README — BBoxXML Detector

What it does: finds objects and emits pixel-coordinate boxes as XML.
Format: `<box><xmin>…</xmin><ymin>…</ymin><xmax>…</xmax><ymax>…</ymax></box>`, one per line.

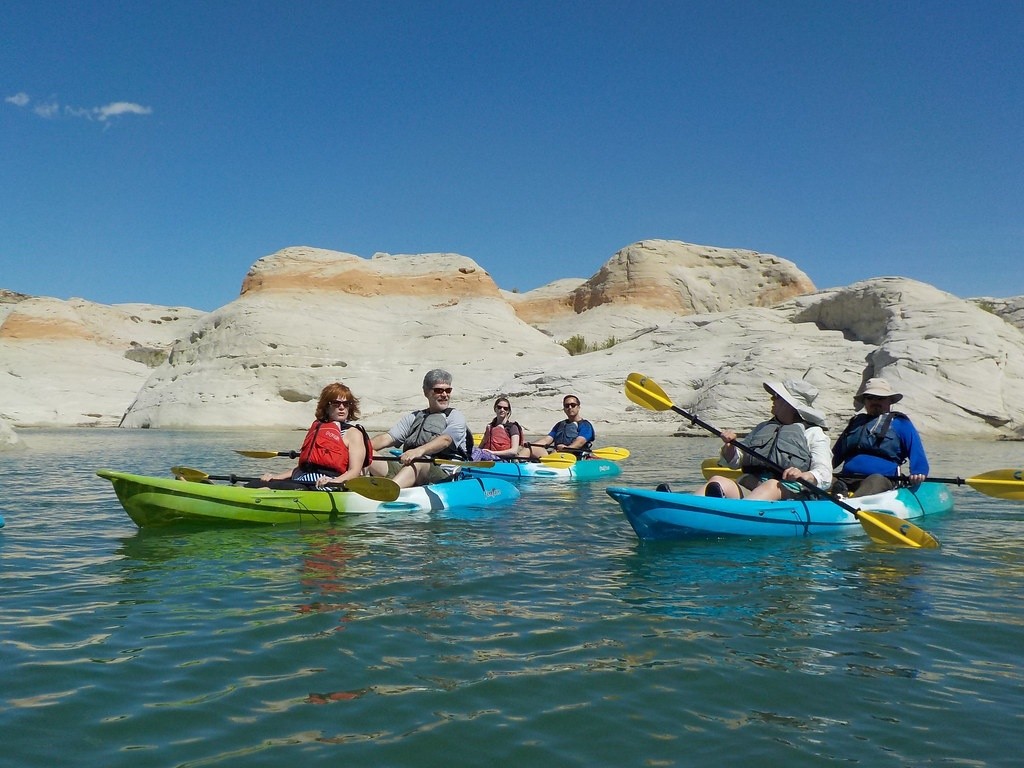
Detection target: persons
<box><xmin>656</xmin><ymin>378</ymin><xmax>833</xmax><ymax>500</ymax></box>
<box><xmin>826</xmin><ymin>379</ymin><xmax>929</xmax><ymax>499</ymax></box>
<box><xmin>174</xmin><ymin>381</ymin><xmax>374</xmax><ymax>491</ymax></box>
<box><xmin>361</xmin><ymin>367</ymin><xmax>467</xmax><ymax>489</ymax></box>
<box><xmin>512</xmin><ymin>394</ymin><xmax>595</xmax><ymax>463</ymax></box>
<box><xmin>465</xmin><ymin>398</ymin><xmax>525</xmax><ymax>462</ymax></box>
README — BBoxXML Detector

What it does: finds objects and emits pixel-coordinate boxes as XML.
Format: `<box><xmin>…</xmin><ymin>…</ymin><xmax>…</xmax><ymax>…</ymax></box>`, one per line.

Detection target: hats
<box><xmin>763</xmin><ymin>377</ymin><xmax>829</xmax><ymax>431</ymax></box>
<box><xmin>854</xmin><ymin>378</ymin><xmax>903</xmax><ymax>412</ymax></box>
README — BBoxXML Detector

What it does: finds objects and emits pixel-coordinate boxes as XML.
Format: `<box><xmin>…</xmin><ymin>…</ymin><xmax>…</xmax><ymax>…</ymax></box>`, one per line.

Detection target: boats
<box><xmin>96</xmin><ymin>463</ymin><xmax>523</xmax><ymax>532</ymax></box>
<box><xmin>604</xmin><ymin>477</ymin><xmax>955</xmax><ymax>543</ymax></box>
<box><xmin>389</xmin><ymin>439</ymin><xmax>623</xmax><ymax>483</ymax></box>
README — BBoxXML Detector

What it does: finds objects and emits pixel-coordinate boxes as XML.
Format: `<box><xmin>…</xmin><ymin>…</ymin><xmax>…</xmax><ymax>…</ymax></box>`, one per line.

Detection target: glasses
<box><xmin>497</xmin><ymin>405</ymin><xmax>508</xmax><ymax>410</ymax></box>
<box><xmin>863</xmin><ymin>394</ymin><xmax>888</xmax><ymax>400</ymax></box>
<box><xmin>429</xmin><ymin>388</ymin><xmax>452</xmax><ymax>394</ymax></box>
<box><xmin>327</xmin><ymin>400</ymin><xmax>353</xmax><ymax>407</ymax></box>
<box><xmin>563</xmin><ymin>403</ymin><xmax>578</xmax><ymax>408</ymax></box>
<box><xmin>774</xmin><ymin>393</ymin><xmax>779</xmax><ymax>399</ymax></box>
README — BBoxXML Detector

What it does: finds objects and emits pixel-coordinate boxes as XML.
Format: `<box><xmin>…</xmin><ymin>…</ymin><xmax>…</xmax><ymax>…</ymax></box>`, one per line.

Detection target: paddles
<box><xmin>624</xmin><ymin>371</ymin><xmax>938</xmax><ymax>547</ymax></box>
<box><xmin>700</xmin><ymin>459</ymin><xmax>1024</xmax><ymax>501</ymax></box>
<box><xmin>229</xmin><ymin>449</ymin><xmax>495</xmax><ymax>468</ymax></box>
<box><xmin>170</xmin><ymin>467</ymin><xmax>400</xmax><ymax>502</ymax></box>
<box><xmin>471</xmin><ymin>433</ymin><xmax>631</xmax><ymax>461</ymax></box>
<box><xmin>487</xmin><ymin>452</ymin><xmax>577</xmax><ymax>468</ymax></box>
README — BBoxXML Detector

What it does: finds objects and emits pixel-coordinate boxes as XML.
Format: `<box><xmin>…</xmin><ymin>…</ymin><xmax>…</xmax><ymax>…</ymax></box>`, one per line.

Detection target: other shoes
<box><xmin>705</xmin><ymin>482</ymin><xmax>726</xmax><ymax>498</ymax></box>
<box><xmin>656</xmin><ymin>483</ymin><xmax>671</xmax><ymax>493</ymax></box>
<box><xmin>831</xmin><ymin>480</ymin><xmax>848</xmax><ymax>500</ymax></box>
